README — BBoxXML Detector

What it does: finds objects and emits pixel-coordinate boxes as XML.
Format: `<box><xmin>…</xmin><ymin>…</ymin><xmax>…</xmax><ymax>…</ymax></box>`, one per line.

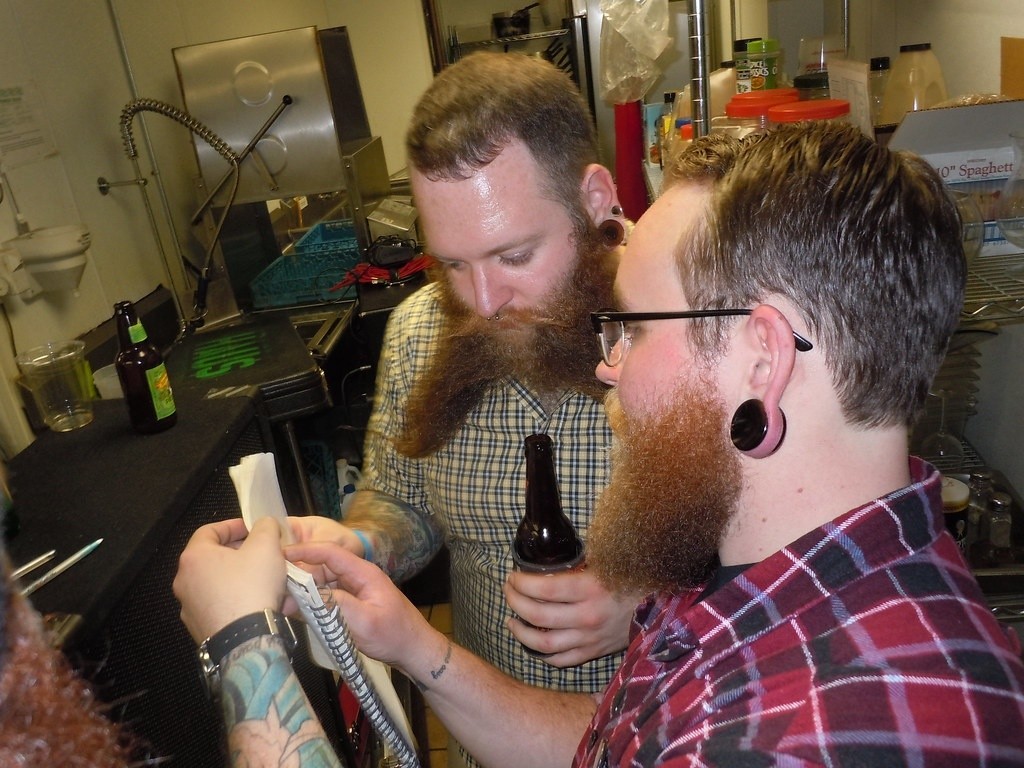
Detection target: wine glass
<box><xmin>919</xmin><ymin>383</ymin><xmax>967</xmax><ymax>472</ymax></box>
<box><xmin>994</xmin><ymin>131</ymin><xmax>1024</xmax><ymax>282</ymax></box>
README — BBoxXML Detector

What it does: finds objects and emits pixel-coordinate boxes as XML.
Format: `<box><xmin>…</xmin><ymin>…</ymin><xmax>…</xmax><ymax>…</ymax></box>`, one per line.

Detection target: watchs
<box><xmin>194</xmin><ymin>607</ymin><xmax>297</xmax><ymax>704</ymax></box>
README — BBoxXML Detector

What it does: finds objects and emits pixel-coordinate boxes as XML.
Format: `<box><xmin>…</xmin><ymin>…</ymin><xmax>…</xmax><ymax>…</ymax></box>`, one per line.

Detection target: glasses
<box><xmin>590</xmin><ymin>307</ymin><xmax>814</xmax><ymax>367</ymax></box>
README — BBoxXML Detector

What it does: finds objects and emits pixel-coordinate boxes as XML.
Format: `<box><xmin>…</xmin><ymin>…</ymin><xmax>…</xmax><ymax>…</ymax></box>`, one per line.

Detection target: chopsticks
<box><xmin>9</xmin><ymin>538</ymin><xmax>103</xmax><ymax>597</ymax></box>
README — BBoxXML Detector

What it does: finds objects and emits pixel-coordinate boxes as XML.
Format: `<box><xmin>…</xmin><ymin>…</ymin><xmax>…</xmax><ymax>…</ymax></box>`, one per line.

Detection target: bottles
<box><xmin>867</xmin><ymin>57</ymin><xmax>893</xmax><ymax>126</ymax></box>
<box><xmin>677</xmin><ymin>124</ymin><xmax>693</xmax><ymax>160</ymax></box>
<box><xmin>672</xmin><ymin>119</ymin><xmax>696</xmax><ymax>166</ymax></box>
<box><xmin>113</xmin><ymin>300</ymin><xmax>177</xmax><ymax>435</ymax></box>
<box><xmin>881</xmin><ymin>42</ymin><xmax>949</xmax><ymax>125</ymax></box>
<box><xmin>966</xmin><ymin>469</ymin><xmax>995</xmax><ymax>524</ymax></box>
<box><xmin>511</xmin><ymin>434</ymin><xmax>586</xmax><ymax>660</ymax></box>
<box><xmin>656</xmin><ymin>90</ymin><xmax>677</xmax><ymax>171</ymax></box>
<box><xmin>983</xmin><ymin>492</ymin><xmax>1012</xmax><ymax>548</ymax></box>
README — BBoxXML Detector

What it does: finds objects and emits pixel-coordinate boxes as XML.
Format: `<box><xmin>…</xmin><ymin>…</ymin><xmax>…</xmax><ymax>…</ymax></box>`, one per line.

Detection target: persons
<box><xmin>256</xmin><ymin>49</ymin><xmax>653</xmax><ymax>768</ymax></box>
<box><xmin>171</xmin><ymin>117</ymin><xmax>1024</xmax><ymax>768</ymax></box>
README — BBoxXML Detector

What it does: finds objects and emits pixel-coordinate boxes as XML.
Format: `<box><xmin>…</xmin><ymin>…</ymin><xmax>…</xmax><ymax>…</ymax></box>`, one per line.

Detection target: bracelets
<box><xmin>352</xmin><ymin>530</ymin><xmax>374</xmax><ymax>561</ymax></box>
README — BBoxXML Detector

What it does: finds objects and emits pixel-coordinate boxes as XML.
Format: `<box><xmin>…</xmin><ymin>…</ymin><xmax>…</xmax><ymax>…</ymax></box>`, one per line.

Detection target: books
<box><xmin>226</xmin><ymin>453</ymin><xmax>421</xmax><ymax>767</ymax></box>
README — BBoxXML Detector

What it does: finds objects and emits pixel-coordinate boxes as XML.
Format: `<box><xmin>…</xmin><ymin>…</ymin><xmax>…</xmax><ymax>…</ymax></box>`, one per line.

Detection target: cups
<box><xmin>14</xmin><ymin>340</ymin><xmax>94</xmax><ymax>432</ymax></box>
<box><xmin>946</xmin><ymin>186</ymin><xmax>986</xmax><ymax>263</ymax></box>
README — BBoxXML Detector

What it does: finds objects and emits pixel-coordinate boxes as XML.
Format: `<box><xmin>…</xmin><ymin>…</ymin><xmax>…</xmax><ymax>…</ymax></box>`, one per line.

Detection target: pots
<box><xmin>491</xmin><ymin>2</ymin><xmax>540</xmax><ymax>37</ymax></box>
<box><xmin>527</xmin><ymin>37</ymin><xmax>577</xmax><ymax>80</ymax></box>
